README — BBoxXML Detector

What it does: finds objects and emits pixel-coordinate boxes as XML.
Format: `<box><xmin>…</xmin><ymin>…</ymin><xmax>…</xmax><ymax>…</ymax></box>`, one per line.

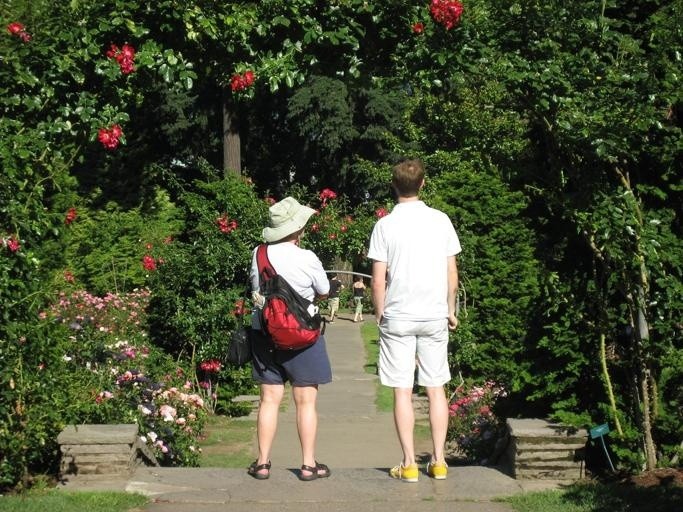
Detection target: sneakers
<box><xmin>389</xmin><ymin>463</ymin><xmax>418</xmax><ymax>483</ymax></box>
<box><xmin>425</xmin><ymin>462</ymin><xmax>447</xmax><ymax>481</ymax></box>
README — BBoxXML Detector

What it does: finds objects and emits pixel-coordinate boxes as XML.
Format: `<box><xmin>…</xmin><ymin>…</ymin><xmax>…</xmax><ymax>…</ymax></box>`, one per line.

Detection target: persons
<box><xmin>350</xmin><ymin>276</ymin><xmax>366</xmax><ymax>323</ymax></box>
<box><xmin>327</xmin><ymin>273</ymin><xmax>345</xmax><ymax>324</ymax></box>
<box><xmin>367</xmin><ymin>159</ymin><xmax>463</xmax><ymax>482</ymax></box>
<box><xmin>247</xmin><ymin>196</ymin><xmax>332</xmax><ymax>481</ymax></box>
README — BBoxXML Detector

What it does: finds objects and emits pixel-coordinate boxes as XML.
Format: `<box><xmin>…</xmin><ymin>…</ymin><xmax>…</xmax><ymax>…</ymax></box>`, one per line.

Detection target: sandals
<box><xmin>301</xmin><ymin>461</ymin><xmax>331</xmax><ymax>481</ymax></box>
<box><xmin>248</xmin><ymin>459</ymin><xmax>271</xmax><ymax>480</ymax></box>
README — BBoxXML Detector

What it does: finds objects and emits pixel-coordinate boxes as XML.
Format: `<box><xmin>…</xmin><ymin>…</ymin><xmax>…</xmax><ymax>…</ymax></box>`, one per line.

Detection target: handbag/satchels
<box><xmin>258</xmin><ymin>275</ymin><xmax>320</xmax><ymax>351</ymax></box>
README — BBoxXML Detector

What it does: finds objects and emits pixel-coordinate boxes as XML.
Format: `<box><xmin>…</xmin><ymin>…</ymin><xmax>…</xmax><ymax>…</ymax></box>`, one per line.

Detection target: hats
<box><xmin>261</xmin><ymin>196</ymin><xmax>317</xmax><ymax>242</ymax></box>
